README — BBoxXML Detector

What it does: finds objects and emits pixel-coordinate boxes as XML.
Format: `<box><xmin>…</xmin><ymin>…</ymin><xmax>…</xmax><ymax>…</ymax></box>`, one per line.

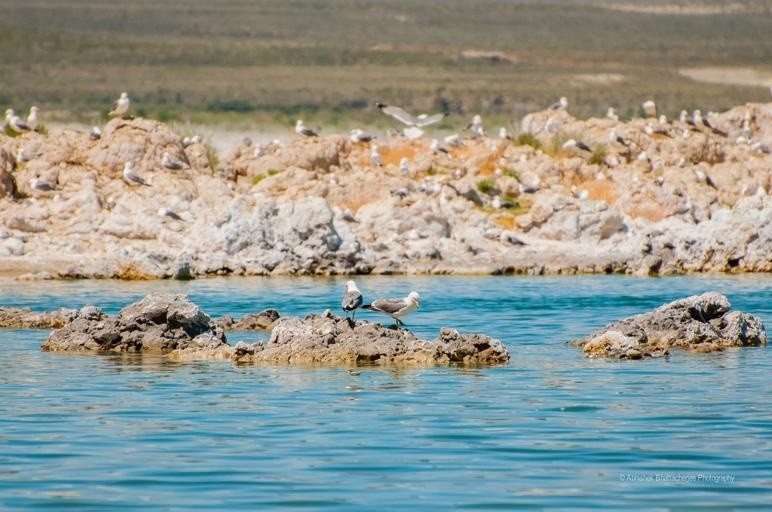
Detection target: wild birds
<box><xmin>341</xmin><ymin>279</ymin><xmax>362</xmax><ymax>324</ymax></box>
<box><xmin>5</xmin><ymin>91</ymin><xmax>770</xmax><ymax>223</ymax></box>
<box><xmin>358</xmin><ymin>290</ymin><xmax>422</xmax><ymax>326</ymax></box>
